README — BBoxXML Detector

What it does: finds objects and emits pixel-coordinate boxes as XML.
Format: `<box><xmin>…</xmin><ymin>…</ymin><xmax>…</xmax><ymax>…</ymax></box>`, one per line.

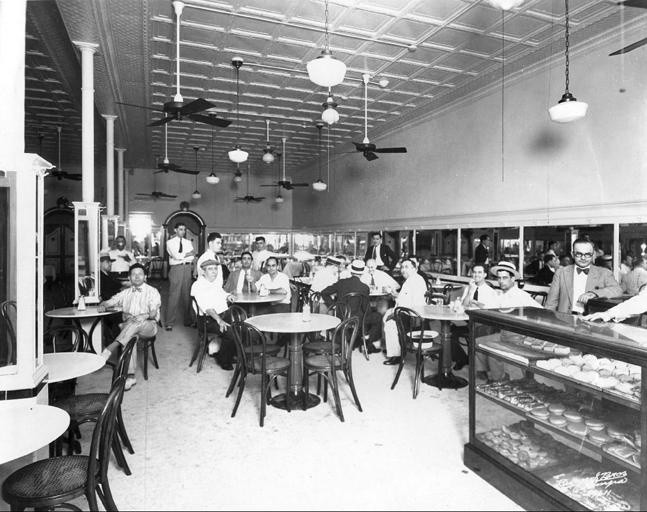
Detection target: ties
<box><xmin>242</xmin><ymin>274</ymin><xmax>248</xmax><ymax>295</ymax></box>
<box><xmin>474</xmin><ymin>288</ymin><xmax>478</xmax><ymax>300</ymax></box>
<box><xmin>373</xmin><ymin>247</ymin><xmax>376</xmax><ymax>258</ymax></box>
<box><xmin>179</xmin><ymin>240</ymin><xmax>182</xmax><ymax>252</ymax></box>
<box><xmin>371</xmin><ymin>274</ymin><xmax>374</xmax><ymax>285</ymax></box>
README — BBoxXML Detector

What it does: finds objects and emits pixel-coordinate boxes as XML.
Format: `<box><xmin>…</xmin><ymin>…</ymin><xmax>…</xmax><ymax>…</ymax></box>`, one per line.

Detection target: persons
<box><xmin>96</xmin><ymin>262</ymin><xmax>162</xmax><ymax>392</ymax></box>
<box><xmin>190</xmin><ymin>232</ymin><xmax>647</xmax><ymax>403</ymax></box>
<box><xmin>109</xmin><ymin>235</ymin><xmax>137</xmax><ymax>275</ymax></box>
<box><xmin>164</xmin><ymin>221</ymin><xmax>195</xmax><ymax>331</ymax></box>
<box><xmin>472</xmin><ymin>234</ymin><xmax>647</xmax><ymax>294</ymax></box>
<box><xmin>99</xmin><ymin>251</ymin><xmax>123</xmax><ymax>301</ymax></box>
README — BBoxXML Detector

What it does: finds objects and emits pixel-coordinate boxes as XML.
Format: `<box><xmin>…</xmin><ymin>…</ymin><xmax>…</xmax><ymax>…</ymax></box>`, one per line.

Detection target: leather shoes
<box><xmin>125</xmin><ymin>378</ymin><xmax>137</xmax><ymax>391</ymax></box>
<box><xmin>220</xmin><ymin>363</ymin><xmax>233</xmax><ymax>370</ymax></box>
<box><xmin>368</xmin><ymin>348</ymin><xmax>510</xmax><ymax>383</ymax></box>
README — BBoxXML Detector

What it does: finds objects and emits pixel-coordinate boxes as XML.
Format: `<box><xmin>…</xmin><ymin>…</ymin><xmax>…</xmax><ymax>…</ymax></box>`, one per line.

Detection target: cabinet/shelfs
<box><xmin>462</xmin><ymin>323</ymin><xmax>647</xmax><ymax>512</ymax></box>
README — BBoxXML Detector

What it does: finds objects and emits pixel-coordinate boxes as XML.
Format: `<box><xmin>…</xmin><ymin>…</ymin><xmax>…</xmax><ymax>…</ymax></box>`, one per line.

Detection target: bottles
<box><xmin>303</xmin><ymin>303</ymin><xmax>312</xmax><ymax>322</ymax></box>
<box><xmin>77</xmin><ymin>295</ymin><xmax>86</xmax><ymax>311</ymax></box>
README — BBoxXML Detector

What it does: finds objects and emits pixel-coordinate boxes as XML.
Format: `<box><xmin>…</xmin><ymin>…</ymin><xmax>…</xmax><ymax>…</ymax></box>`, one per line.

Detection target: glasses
<box><xmin>573</xmin><ymin>252</ymin><xmax>594</xmax><ymax>258</ymax></box>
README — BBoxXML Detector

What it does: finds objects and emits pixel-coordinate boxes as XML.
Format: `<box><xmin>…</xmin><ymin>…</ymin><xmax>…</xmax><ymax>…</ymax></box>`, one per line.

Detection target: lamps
<box><xmin>485</xmin><ymin>1</ymin><xmax>588</xmax><ymax>123</ymax></box>
<box><xmin>306</xmin><ymin>0</ymin><xmax>346</xmax><ymax>191</ymax></box>
<box><xmin>191</xmin><ymin>128</ymin><xmax>220</xmax><ymax>199</ymax></box>
<box><xmin>227</xmin><ymin>56</ymin><xmax>276</xmax><ymax>164</ymax></box>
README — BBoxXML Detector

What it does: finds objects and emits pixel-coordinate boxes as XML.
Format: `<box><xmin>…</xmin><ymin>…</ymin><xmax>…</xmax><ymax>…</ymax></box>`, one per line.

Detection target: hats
<box><xmin>100</xmin><ymin>252</ymin><xmax>116</xmax><ymax>262</ymax></box>
<box><xmin>491</xmin><ymin>261</ymin><xmax>521</xmax><ymax>281</ymax></box>
<box><xmin>200</xmin><ymin>260</ymin><xmax>221</xmax><ymax>266</ymax></box>
<box><xmin>326</xmin><ymin>256</ymin><xmax>342</xmax><ymax>266</ymax></box>
<box><xmin>347</xmin><ymin>259</ymin><xmax>369</xmax><ymax>274</ymax></box>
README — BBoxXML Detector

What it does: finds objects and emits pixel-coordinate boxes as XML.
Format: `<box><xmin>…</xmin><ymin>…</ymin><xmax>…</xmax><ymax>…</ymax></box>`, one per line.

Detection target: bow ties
<box><xmin>132</xmin><ymin>287</ymin><xmax>142</xmax><ymax>292</ymax></box>
<box><xmin>577</xmin><ymin>267</ymin><xmax>590</xmax><ymax>274</ymax></box>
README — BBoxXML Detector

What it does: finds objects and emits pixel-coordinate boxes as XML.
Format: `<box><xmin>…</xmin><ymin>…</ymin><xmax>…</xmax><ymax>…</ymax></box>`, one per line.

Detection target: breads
<box><xmin>530</xmin><ymin>399</ymin><xmax>642</xmax><ymax>469</ymax></box>
<box><xmin>544</xmin><ymin>466</ymin><xmax>644</xmax><ymax>512</ymax></box>
<box><xmin>473</xmin><ymin>422</ymin><xmax>581</xmax><ymax>471</ymax></box>
<box><xmin>475</xmin><ymin>376</ymin><xmax>567</xmax><ymax>411</ymax></box>
<box><xmin>500</xmin><ymin>329</ymin><xmax>571</xmax><ymax>356</ymax></box>
<box><xmin>535</xmin><ymin>354</ymin><xmax>642</xmax><ymax>399</ymax></box>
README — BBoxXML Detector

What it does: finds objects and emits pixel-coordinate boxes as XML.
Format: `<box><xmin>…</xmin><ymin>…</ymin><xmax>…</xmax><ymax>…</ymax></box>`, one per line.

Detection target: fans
<box><xmin>610</xmin><ymin>1</ymin><xmax>647</xmax><ymax>55</ymax></box>
<box><xmin>112</xmin><ymin>1</ymin><xmax>233</xmax><ymax>128</ymax></box>
<box><xmin>260</xmin><ymin>136</ymin><xmax>309</xmax><ymax>191</ymax></box>
<box><xmin>135</xmin><ymin>191</ymin><xmax>177</xmax><ymax>199</ymax></box>
<box><xmin>234</xmin><ymin>164</ymin><xmax>266</xmax><ymax>202</ymax></box>
<box><xmin>333</xmin><ymin>73</ymin><xmax>408</xmax><ymax>160</ymax></box>
<box><xmin>151</xmin><ymin>112</ymin><xmax>200</xmax><ymax>175</ymax></box>
<box><xmin>50</xmin><ymin>124</ymin><xmax>82</xmax><ymax>180</ymax></box>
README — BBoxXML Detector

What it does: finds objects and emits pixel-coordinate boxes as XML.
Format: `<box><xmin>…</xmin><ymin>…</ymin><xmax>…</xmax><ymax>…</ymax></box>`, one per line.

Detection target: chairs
<box><xmin>1</xmin><ymin>299</ymin><xmax>159</xmax><ymax>511</ymax></box>
